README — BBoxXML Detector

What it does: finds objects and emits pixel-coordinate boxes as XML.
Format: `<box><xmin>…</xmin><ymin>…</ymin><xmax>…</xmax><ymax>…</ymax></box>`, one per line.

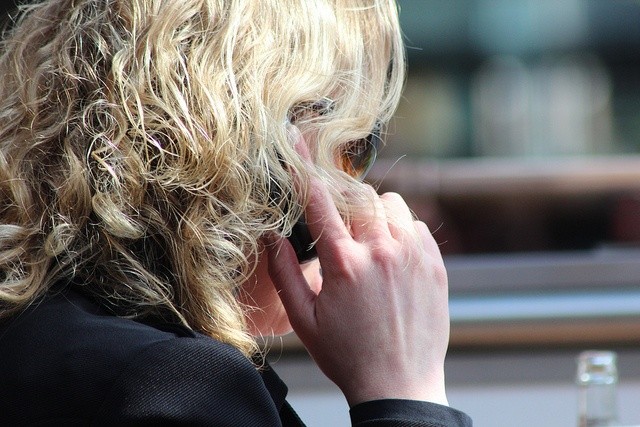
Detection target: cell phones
<box><xmin>242</xmin><ymin>144</ymin><xmax>318</xmax><ymax>271</ymax></box>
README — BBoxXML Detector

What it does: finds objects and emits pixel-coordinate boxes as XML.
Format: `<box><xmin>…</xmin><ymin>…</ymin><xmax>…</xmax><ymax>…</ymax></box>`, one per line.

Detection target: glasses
<box><xmin>339</xmin><ymin>132</ymin><xmax>378</xmax><ymax>180</ymax></box>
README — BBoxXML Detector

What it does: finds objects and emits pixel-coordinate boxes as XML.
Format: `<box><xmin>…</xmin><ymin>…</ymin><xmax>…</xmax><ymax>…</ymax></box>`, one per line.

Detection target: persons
<box><xmin>0</xmin><ymin>0</ymin><xmax>472</xmax><ymax>427</ymax></box>
